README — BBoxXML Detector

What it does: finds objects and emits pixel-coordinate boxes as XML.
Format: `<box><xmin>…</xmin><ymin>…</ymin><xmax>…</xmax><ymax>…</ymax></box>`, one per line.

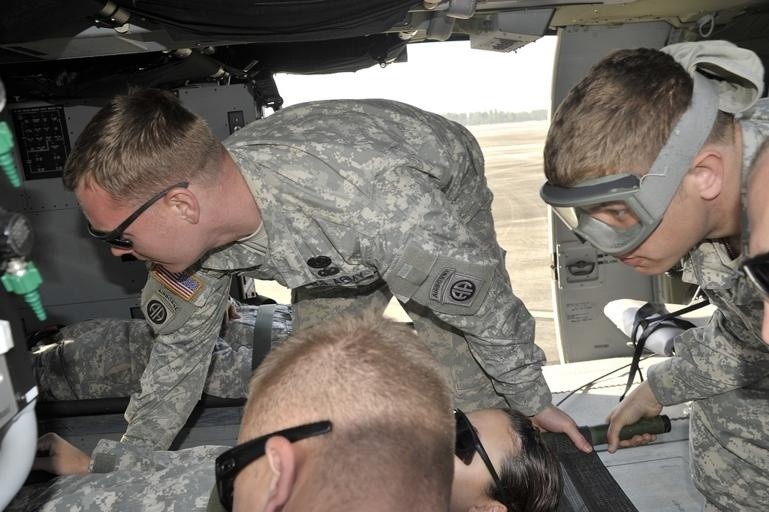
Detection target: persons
<box><xmin>746</xmin><ymin>136</ymin><xmax>769</xmax><ymax>346</ymax></box>
<box><xmin>230</xmin><ymin>312</ymin><xmax>455</xmax><ymax>512</ymax></box>
<box><xmin>543</xmin><ymin>48</ymin><xmax>769</xmax><ymax>512</ymax></box>
<box><xmin>28</xmin><ymin>294</ymin><xmax>297</xmax><ymax>402</ymax></box>
<box><xmin>60</xmin><ymin>86</ymin><xmax>592</xmax><ymax>454</ymax></box>
<box><xmin>2</xmin><ymin>409</ymin><xmax>563</xmax><ymax>512</ymax></box>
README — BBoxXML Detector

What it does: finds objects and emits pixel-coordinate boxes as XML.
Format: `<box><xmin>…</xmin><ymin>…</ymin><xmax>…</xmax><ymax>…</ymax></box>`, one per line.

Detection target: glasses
<box><xmin>215</xmin><ymin>421</ymin><xmax>332</xmax><ymax>512</ymax></box>
<box><xmin>455</xmin><ymin>408</ymin><xmax>508</xmax><ymax>502</ymax></box>
<box><xmin>85</xmin><ymin>182</ymin><xmax>190</xmax><ymax>252</ymax></box>
<box><xmin>540</xmin><ymin>129</ymin><xmax>710</xmax><ymax>260</ymax></box>
